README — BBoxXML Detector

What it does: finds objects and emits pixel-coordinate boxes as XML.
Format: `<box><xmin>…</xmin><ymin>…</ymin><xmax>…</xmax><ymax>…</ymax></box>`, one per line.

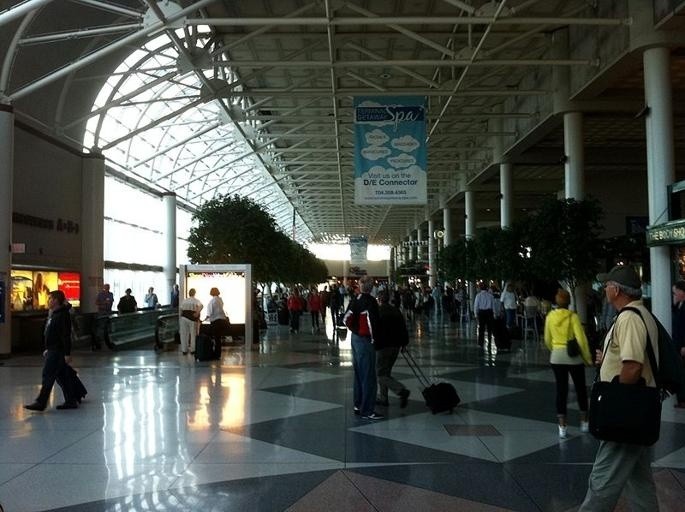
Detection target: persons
<box><xmin>171</xmin><ymin>284</ymin><xmax>179</xmax><ymax>309</ymax></box>
<box><xmin>672</xmin><ymin>283</ymin><xmax>685</xmax><ymax>408</ymax></box>
<box><xmin>145</xmin><ymin>287</ymin><xmax>158</xmax><ymax>307</ymax></box>
<box><xmin>95</xmin><ymin>284</ymin><xmax>114</xmax><ymax>313</ymax></box>
<box><xmin>577</xmin><ymin>263</ymin><xmax>660</xmax><ymax>511</ymax></box>
<box><xmin>178</xmin><ymin>289</ymin><xmax>203</xmax><ymax>355</ymax></box>
<box><xmin>252</xmin><ymin>264</ymin><xmax>618</xmax><ymax>439</ymax></box>
<box><xmin>23</xmin><ymin>290</ymin><xmax>87</xmax><ymax>411</ymax></box>
<box><xmin>117</xmin><ymin>289</ymin><xmax>137</xmax><ymax>314</ymax></box>
<box><xmin>200</xmin><ymin>288</ymin><xmax>227</xmax><ymax>360</ymax></box>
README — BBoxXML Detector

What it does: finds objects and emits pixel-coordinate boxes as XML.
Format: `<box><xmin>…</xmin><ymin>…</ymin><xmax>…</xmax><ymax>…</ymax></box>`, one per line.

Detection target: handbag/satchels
<box><xmin>589</xmin><ymin>375</ymin><xmax>661</xmax><ymax>446</ymax></box>
<box><xmin>567</xmin><ymin>339</ymin><xmax>580</xmax><ymax>358</ymax></box>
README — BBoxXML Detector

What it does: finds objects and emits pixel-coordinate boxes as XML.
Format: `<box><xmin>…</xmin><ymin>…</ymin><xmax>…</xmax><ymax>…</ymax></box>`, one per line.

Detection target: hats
<box><xmin>596</xmin><ymin>266</ymin><xmax>640</xmax><ymax>288</ymax></box>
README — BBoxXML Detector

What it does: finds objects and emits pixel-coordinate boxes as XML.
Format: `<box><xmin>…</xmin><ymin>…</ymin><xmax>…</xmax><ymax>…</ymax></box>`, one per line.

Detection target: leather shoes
<box><xmin>24</xmin><ymin>403</ymin><xmax>45</xmax><ymax>411</ymax></box>
<box><xmin>56</xmin><ymin>402</ymin><xmax>77</xmax><ymax>409</ymax></box>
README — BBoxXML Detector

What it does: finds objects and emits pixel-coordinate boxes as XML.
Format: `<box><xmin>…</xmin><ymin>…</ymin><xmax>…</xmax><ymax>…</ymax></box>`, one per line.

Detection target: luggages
<box><xmin>195</xmin><ymin>334</ymin><xmax>212</xmax><ymax>361</ymax></box>
<box><xmin>57</xmin><ymin>365</ymin><xmax>87</xmax><ymax>404</ymax></box>
<box><xmin>401</xmin><ymin>349</ymin><xmax>460</xmax><ymax>413</ymax></box>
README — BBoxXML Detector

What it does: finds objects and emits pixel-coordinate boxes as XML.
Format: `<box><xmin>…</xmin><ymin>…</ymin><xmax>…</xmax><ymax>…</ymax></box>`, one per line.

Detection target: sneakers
<box><xmin>559</xmin><ymin>421</ymin><xmax>588</xmax><ymax>438</ymax></box>
<box><xmin>353</xmin><ymin>390</ymin><xmax>410</xmax><ymax>420</ymax></box>
